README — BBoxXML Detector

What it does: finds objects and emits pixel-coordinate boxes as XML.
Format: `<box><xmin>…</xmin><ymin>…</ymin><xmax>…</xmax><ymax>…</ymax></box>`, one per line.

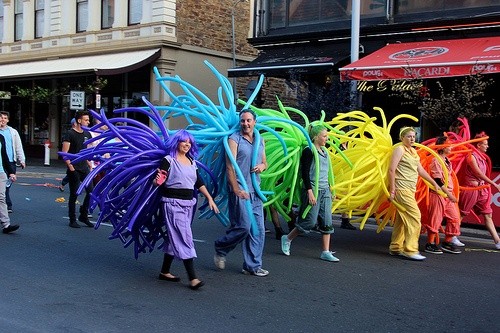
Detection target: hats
<box><xmin>71</xmin><ymin>118</ymin><xmax>76</xmax><ymax>124</ymax></box>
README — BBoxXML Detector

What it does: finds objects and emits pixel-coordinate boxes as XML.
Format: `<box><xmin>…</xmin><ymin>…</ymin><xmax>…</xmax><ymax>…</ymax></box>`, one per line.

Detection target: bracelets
<box><xmin>391</xmin><ymin>192</ymin><xmax>395</xmax><ymax>194</ymax></box>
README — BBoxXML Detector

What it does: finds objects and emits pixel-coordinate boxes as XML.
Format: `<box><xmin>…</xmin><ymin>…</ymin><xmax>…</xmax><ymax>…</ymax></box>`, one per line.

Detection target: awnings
<box><xmin>0</xmin><ymin>47</ymin><xmax>161</xmax><ymax>79</ymax></box>
<box><xmin>338</xmin><ymin>37</ymin><xmax>500</xmax><ymax>82</ymax></box>
<box><xmin>227</xmin><ymin>41</ymin><xmax>351</xmax><ymax>78</ymax></box>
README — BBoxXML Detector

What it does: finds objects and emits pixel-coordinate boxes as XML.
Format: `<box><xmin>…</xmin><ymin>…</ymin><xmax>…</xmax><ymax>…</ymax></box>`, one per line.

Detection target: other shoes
<box><xmin>8</xmin><ymin>209</ymin><xmax>12</xmax><ymax>213</ymax></box>
<box><xmin>79</xmin><ymin>215</ymin><xmax>94</xmax><ymax>227</ymax></box>
<box><xmin>69</xmin><ymin>221</ymin><xmax>80</xmax><ymax>228</ymax></box>
<box><xmin>158</xmin><ymin>274</ymin><xmax>180</xmax><ymax>282</ymax></box>
<box><xmin>341</xmin><ymin>221</ymin><xmax>356</xmax><ymax>230</ymax></box>
<box><xmin>276</xmin><ymin>226</ymin><xmax>288</xmax><ymax>240</ymax></box>
<box><xmin>389</xmin><ymin>251</ymin><xmax>404</xmax><ymax>256</ymax></box>
<box><xmin>403</xmin><ymin>251</ymin><xmax>426</xmax><ymax>260</ymax></box>
<box><xmin>61</xmin><ymin>183</ymin><xmax>65</xmax><ymax>192</ymax></box>
<box><xmin>189</xmin><ymin>281</ymin><xmax>204</xmax><ymax>289</ymax></box>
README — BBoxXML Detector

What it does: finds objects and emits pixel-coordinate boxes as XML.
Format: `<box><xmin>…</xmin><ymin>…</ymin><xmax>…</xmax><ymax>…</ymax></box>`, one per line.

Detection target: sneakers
<box><xmin>241</xmin><ymin>267</ymin><xmax>269</xmax><ymax>277</ymax></box>
<box><xmin>2</xmin><ymin>224</ymin><xmax>19</xmax><ymax>233</ymax></box>
<box><xmin>441</xmin><ymin>242</ymin><xmax>462</xmax><ymax>253</ymax></box>
<box><xmin>281</xmin><ymin>234</ymin><xmax>292</xmax><ymax>255</ymax></box>
<box><xmin>320</xmin><ymin>250</ymin><xmax>340</xmax><ymax>262</ymax></box>
<box><xmin>494</xmin><ymin>241</ymin><xmax>500</xmax><ymax>248</ymax></box>
<box><xmin>451</xmin><ymin>237</ymin><xmax>465</xmax><ymax>246</ymax></box>
<box><xmin>310</xmin><ymin>225</ymin><xmax>321</xmax><ymax>233</ymax></box>
<box><xmin>424</xmin><ymin>243</ymin><xmax>443</xmax><ymax>254</ymax></box>
<box><xmin>214</xmin><ymin>245</ymin><xmax>225</xmax><ymax>269</ymax></box>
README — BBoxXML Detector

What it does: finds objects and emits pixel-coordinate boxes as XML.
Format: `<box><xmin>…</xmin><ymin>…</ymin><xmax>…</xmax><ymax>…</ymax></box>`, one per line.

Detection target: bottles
<box><xmin>6</xmin><ymin>176</ymin><xmax>14</xmax><ymax>188</ymax></box>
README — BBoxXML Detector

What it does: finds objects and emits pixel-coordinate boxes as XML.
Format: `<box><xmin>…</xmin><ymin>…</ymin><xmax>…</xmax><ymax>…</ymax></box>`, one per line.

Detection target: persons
<box><xmin>0</xmin><ymin>111</ymin><xmax>25</xmax><ymax>213</ymax></box>
<box><xmin>281</xmin><ymin>125</ymin><xmax>340</xmax><ymax>261</ymax></box>
<box><xmin>263</xmin><ymin>175</ymin><xmax>297</xmax><ymax>240</ymax></box>
<box><xmin>387</xmin><ymin>127</ymin><xmax>441</xmax><ymax>260</ymax></box>
<box><xmin>59</xmin><ymin>118</ymin><xmax>77</xmax><ymax>192</ymax></box>
<box><xmin>340</xmin><ymin>212</ymin><xmax>357</xmax><ymax>230</ymax></box>
<box><xmin>88</xmin><ymin>118</ymin><xmax>131</xmax><ymax>218</ymax></box>
<box><xmin>451</xmin><ymin>132</ymin><xmax>500</xmax><ymax>249</ymax></box>
<box><xmin>0</xmin><ymin>112</ymin><xmax>20</xmax><ymax>233</ymax></box>
<box><xmin>214</xmin><ymin>109</ymin><xmax>269</xmax><ymax>277</ymax></box>
<box><xmin>62</xmin><ymin>110</ymin><xmax>94</xmax><ymax>228</ymax></box>
<box><xmin>153</xmin><ymin>129</ymin><xmax>220</xmax><ymax>291</ymax></box>
<box><xmin>425</xmin><ymin>136</ymin><xmax>463</xmax><ymax>255</ymax></box>
